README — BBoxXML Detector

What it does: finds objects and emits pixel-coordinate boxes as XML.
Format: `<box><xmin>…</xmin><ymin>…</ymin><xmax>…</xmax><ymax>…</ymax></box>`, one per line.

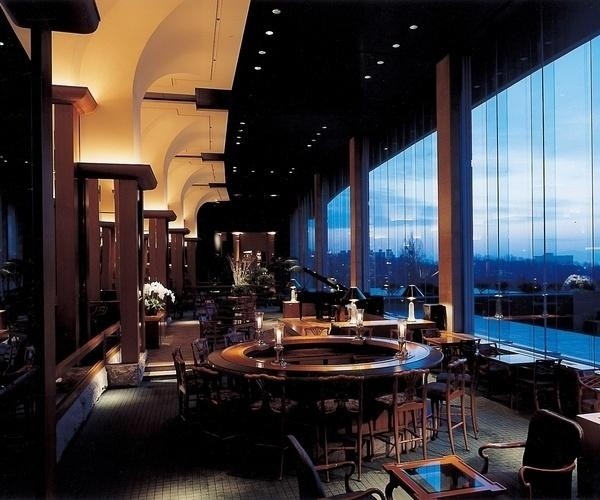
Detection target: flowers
<box><xmin>137</xmin><ymin>281</ymin><xmax>176</xmax><ymax>308</ymax></box>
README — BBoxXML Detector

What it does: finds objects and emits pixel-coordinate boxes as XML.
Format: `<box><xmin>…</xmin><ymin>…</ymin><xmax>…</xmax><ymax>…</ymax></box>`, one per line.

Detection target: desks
<box><xmin>382</xmin><ymin>456</ymin><xmax>507</xmax><ymax>500</ymax></box>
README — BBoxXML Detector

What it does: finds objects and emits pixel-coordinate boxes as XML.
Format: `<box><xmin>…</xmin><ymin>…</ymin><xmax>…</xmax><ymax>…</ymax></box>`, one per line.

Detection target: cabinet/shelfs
<box><xmin>282</xmin><ymin>301</ymin><xmax>300</xmax><ymax>319</ymax></box>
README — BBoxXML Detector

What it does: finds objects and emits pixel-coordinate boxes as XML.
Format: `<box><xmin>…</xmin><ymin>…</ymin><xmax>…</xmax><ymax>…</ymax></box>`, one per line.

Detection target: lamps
<box><xmin>287</xmin><ymin>279</ymin><xmax>303</xmax><ymax>301</ymax></box>
<box><xmin>401</xmin><ymin>285</ymin><xmax>425</xmax><ymax>321</ymax></box>
<box><xmin>342</xmin><ymin>287</ymin><xmax>366</xmax><ymax>323</ymax></box>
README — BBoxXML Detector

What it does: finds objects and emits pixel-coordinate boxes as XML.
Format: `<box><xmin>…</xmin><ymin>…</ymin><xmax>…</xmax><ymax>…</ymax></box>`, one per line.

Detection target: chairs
<box><xmin>242</xmin><ymin>374</ymin><xmax>297</xmax><ymax>482</ymax></box>
<box><xmin>477</xmin><ymin>342</ymin><xmax>512</xmax><ymax>396</ymax></box>
<box><xmin>173</xmin><ymin>347</ymin><xmax>215</xmax><ymax>424</ymax></box>
<box><xmin>304</xmin><ymin>375</ymin><xmax>363</xmax><ymax>482</ymax></box>
<box><xmin>421</xmin><ymin>328</ymin><xmax>440</xmax><ymax>346</ymax></box>
<box><xmin>370</xmin><ymin>370</ymin><xmax>429</xmax><ymax>464</ymax></box>
<box><xmin>287</xmin><ymin>434</ymin><xmax>385</xmax><ymax>500</ymax></box>
<box><xmin>422</xmin><ymin>359</ymin><xmax>472</xmax><ymax>456</ymax></box>
<box><xmin>512</xmin><ymin>358</ymin><xmax>565</xmax><ymax>414</ymax></box>
<box><xmin>448</xmin><ymin>339</ymin><xmax>481</xmax><ymax>385</ymax></box>
<box><xmin>190</xmin><ymin>336</ymin><xmax>223</xmax><ymax>405</ymax></box>
<box><xmin>478</xmin><ymin>409</ymin><xmax>584</xmax><ymax>500</ymax></box>
<box><xmin>434</xmin><ymin>357</ymin><xmax>481</xmax><ymax>440</ymax></box>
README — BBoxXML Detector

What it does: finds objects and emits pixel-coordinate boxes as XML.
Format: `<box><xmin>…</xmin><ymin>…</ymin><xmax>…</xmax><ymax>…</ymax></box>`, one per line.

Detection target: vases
<box><xmin>145</xmin><ymin>304</ymin><xmax>158</xmax><ymax>317</ymax></box>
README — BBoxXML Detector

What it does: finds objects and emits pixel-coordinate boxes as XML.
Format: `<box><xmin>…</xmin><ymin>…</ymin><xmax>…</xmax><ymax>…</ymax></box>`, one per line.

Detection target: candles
<box><xmin>357</xmin><ymin>314</ymin><xmax>362</xmax><ymax>324</ymax></box>
<box><xmin>277</xmin><ymin>331</ymin><xmax>282</xmax><ymax>345</ymax></box>
<box><xmin>258</xmin><ymin>317</ymin><xmax>261</xmax><ymax>329</ymax></box>
<box><xmin>400</xmin><ymin>325</ymin><xmax>405</xmax><ymax>337</ymax></box>
